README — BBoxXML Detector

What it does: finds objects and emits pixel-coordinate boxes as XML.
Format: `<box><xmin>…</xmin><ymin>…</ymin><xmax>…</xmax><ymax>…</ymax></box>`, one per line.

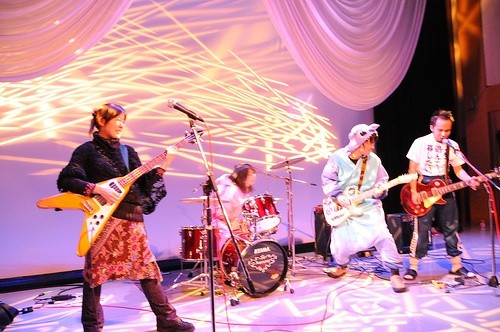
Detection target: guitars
<box><xmin>35</xmin><ymin>127</ymin><xmax>205</xmax><ymax>259</ymax></box>
<box><xmin>399</xmin><ymin>166</ymin><xmax>500</xmax><ymax>217</ymax></box>
<box><xmin>322</xmin><ymin>171</ymin><xmax>420</xmax><ymax>227</ymax></box>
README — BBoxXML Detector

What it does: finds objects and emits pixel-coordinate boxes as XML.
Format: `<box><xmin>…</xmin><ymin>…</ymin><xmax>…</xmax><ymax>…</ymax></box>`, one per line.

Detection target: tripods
<box><xmin>164</xmin><ymin>201</ymin><xmax>240</xmax><ymax>307</ymax></box>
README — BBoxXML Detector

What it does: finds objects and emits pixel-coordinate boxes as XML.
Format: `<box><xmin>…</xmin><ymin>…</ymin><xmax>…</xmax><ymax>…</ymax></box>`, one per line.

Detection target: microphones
<box><xmin>167</xmin><ymin>99</ymin><xmax>205</xmax><ymax>123</ymax></box>
<box><xmin>442</xmin><ymin>139</ymin><xmax>459</xmax><ymax>151</ymax></box>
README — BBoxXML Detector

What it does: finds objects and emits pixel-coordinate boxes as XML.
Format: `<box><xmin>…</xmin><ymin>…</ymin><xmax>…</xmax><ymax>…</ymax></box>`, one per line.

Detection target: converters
<box><xmin>22</xmin><ymin>307</ymin><xmax>33</xmax><ymax>314</ymax></box>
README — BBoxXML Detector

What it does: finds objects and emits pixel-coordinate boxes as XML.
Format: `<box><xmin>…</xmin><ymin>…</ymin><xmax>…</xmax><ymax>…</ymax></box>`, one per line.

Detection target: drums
<box><xmin>241</xmin><ymin>193</ymin><xmax>282</xmax><ymax>234</ymax></box>
<box><xmin>220</xmin><ymin>232</ymin><xmax>289</xmax><ymax>298</ymax></box>
<box><xmin>178</xmin><ymin>226</ymin><xmax>219</xmax><ymax>264</ymax></box>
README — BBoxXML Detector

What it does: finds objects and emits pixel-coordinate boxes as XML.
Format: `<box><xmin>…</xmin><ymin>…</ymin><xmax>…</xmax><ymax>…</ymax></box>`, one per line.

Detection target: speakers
<box><xmin>387</xmin><ymin>214</ymin><xmax>433</xmax><ymax>255</ymax></box>
<box><xmin>314</xmin><ymin>212</ymin><xmax>332</xmax><ymax>256</ymax></box>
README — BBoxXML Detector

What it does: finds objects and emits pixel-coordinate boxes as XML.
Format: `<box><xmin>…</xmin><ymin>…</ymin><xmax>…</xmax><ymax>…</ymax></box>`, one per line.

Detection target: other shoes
<box><xmin>327</xmin><ymin>265</ymin><xmax>348</xmax><ymax>278</ymax></box>
<box><xmin>156</xmin><ymin>318</ymin><xmax>195</xmax><ymax>332</ymax></box>
<box><xmin>390</xmin><ymin>275</ymin><xmax>406</xmax><ymax>293</ymax></box>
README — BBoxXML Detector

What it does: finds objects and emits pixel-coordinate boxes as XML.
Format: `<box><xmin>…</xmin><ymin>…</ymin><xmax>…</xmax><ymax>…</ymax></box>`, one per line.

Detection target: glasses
<box><xmin>359</xmin><ymin>129</ymin><xmax>375</xmax><ymax>136</ymax></box>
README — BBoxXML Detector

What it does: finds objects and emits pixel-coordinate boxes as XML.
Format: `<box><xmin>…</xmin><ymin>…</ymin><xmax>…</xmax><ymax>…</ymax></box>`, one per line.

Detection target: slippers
<box><xmin>448</xmin><ymin>267</ymin><xmax>476</xmax><ymax>280</ymax></box>
<box><xmin>403</xmin><ymin>269</ymin><xmax>418</xmax><ymax>281</ymax></box>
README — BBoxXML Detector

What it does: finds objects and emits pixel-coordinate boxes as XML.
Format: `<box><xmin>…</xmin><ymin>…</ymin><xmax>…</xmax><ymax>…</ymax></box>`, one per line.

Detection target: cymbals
<box><xmin>178</xmin><ymin>196</ymin><xmax>229</xmax><ymax>203</ymax></box>
<box><xmin>269</xmin><ymin>156</ymin><xmax>306</xmax><ymax>171</ymax></box>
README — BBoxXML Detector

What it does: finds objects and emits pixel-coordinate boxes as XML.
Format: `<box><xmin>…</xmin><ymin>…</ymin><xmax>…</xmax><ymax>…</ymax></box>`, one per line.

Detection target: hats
<box><xmin>347</xmin><ymin>123</ymin><xmax>380</xmax><ymax>152</ymax></box>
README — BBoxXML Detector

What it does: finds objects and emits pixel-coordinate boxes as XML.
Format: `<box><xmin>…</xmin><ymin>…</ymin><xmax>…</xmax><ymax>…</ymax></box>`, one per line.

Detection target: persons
<box><xmin>403</xmin><ymin>111</ymin><xmax>482</xmax><ymax>280</ymax></box>
<box><xmin>56</xmin><ymin>103</ymin><xmax>194</xmax><ymax>332</ymax></box>
<box><xmin>218</xmin><ymin>163</ymin><xmax>256</xmax><ymax>237</ymax></box>
<box><xmin>320</xmin><ymin>124</ymin><xmax>405</xmax><ymax>292</ymax></box>
